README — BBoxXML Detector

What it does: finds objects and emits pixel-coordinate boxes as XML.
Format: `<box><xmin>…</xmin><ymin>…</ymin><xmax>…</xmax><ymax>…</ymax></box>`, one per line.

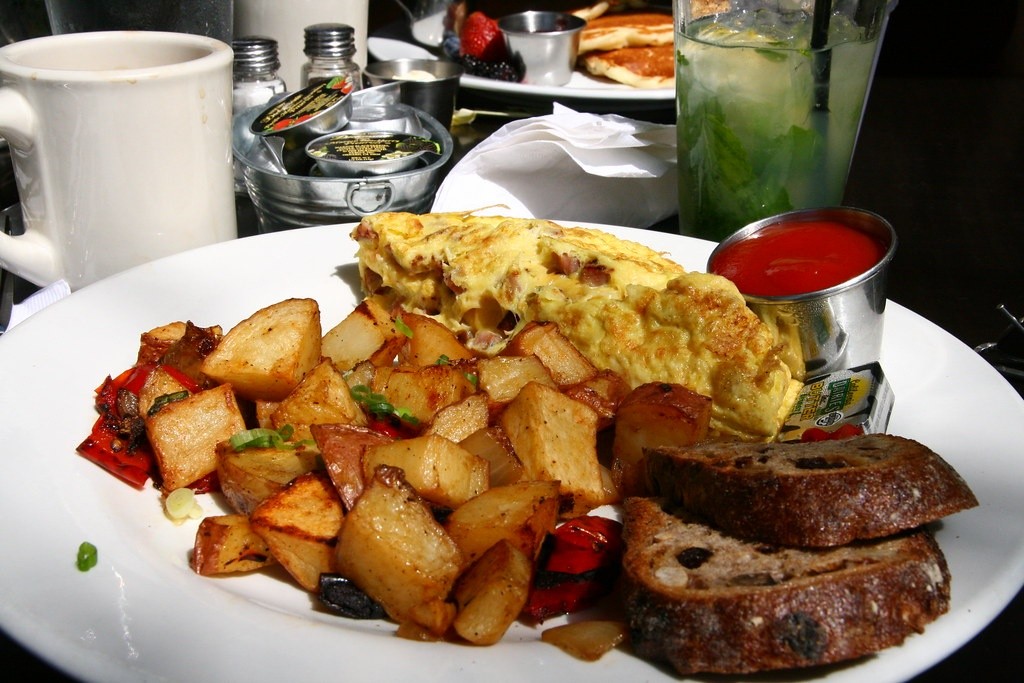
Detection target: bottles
<box><xmin>229</xmin><ymin>38</ymin><xmax>287</xmax><ymax>197</ymax></box>
<box><xmin>301</xmin><ymin>23</ymin><xmax>362</xmax><ymax>93</ymax></box>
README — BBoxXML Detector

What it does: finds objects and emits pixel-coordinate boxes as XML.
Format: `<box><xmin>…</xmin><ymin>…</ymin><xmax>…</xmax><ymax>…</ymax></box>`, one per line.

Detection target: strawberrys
<box><xmin>458</xmin><ymin>11</ymin><xmax>510</xmax><ymax>64</ymax></box>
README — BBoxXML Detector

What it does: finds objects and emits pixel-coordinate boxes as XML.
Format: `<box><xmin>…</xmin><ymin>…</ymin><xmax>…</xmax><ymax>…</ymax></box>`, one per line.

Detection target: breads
<box><xmin>623</xmin><ymin>434</ymin><xmax>980</xmax><ymax>680</ymax></box>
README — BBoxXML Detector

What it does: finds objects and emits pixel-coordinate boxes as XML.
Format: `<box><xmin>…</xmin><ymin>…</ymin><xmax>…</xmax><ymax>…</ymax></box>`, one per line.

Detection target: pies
<box><xmin>578</xmin><ymin>13</ymin><xmax>674</xmax><ymax>88</ymax></box>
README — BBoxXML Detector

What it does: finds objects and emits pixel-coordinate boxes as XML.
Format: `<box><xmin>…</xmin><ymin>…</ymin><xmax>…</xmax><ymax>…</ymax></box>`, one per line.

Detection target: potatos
<box><xmin>139</xmin><ymin>297</ymin><xmax>714</xmax><ymax>659</ymax></box>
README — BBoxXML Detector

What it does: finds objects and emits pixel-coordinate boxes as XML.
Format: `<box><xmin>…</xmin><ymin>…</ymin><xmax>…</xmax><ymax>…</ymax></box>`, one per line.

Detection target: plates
<box><xmin>367</xmin><ymin>36</ymin><xmax>438</xmax><ymax>61</ymax></box>
<box><xmin>0</xmin><ymin>218</ymin><xmax>1024</xmax><ymax>683</ymax></box>
<box><xmin>411</xmin><ymin>11</ymin><xmax>446</xmax><ymax>49</ymax></box>
<box><xmin>460</xmin><ymin>61</ymin><xmax>676</xmax><ymax>119</ymax></box>
<box><xmin>460</xmin><ymin>67</ymin><xmax>676</xmax><ymax>109</ymax></box>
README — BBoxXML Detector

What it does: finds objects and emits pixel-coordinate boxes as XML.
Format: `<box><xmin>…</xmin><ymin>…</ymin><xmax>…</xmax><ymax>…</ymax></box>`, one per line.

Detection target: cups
<box><xmin>0</xmin><ymin>32</ymin><xmax>238</xmax><ymax>293</ymax></box>
<box><xmin>45</xmin><ymin>0</ymin><xmax>235</xmax><ymax>53</ymax></box>
<box><xmin>706</xmin><ymin>204</ymin><xmax>897</xmax><ymax>381</ymax></box>
<box><xmin>672</xmin><ymin>0</ymin><xmax>892</xmax><ymax>242</ymax></box>
<box><xmin>497</xmin><ymin>10</ymin><xmax>587</xmax><ymax>87</ymax></box>
<box><xmin>362</xmin><ymin>61</ymin><xmax>462</xmax><ymax>134</ymax></box>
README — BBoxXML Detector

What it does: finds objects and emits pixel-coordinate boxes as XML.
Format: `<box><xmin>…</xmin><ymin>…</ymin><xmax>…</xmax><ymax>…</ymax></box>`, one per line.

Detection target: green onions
<box><xmin>76</xmin><ymin>314</ymin><xmax>479</xmax><ymax>573</ymax></box>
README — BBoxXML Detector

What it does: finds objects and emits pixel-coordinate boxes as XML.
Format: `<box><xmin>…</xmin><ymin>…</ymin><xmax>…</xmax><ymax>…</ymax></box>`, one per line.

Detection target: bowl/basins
<box><xmin>248</xmin><ymin>76</ymin><xmax>433</xmax><ymax>178</ymax></box>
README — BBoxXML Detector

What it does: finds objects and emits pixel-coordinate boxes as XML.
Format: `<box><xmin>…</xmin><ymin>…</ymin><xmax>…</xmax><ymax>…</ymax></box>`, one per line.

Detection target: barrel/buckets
<box><xmin>232</xmin><ymin>97</ymin><xmax>453</xmax><ymax>234</ymax></box>
